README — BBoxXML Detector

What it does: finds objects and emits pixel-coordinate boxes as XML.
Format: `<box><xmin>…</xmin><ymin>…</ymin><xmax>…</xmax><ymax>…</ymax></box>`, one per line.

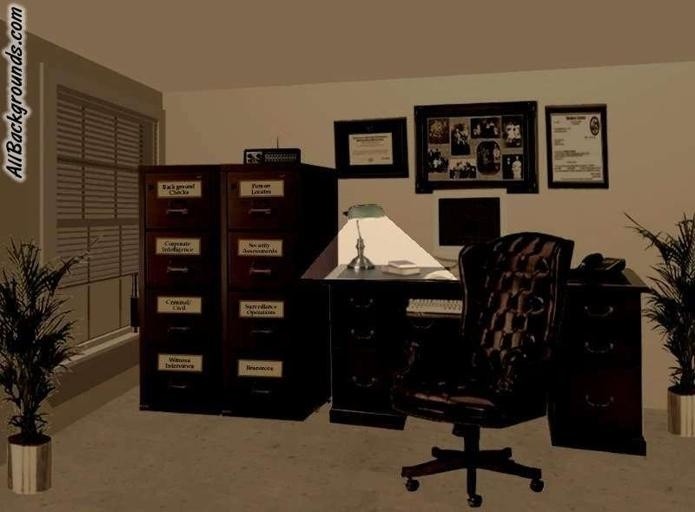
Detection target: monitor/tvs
<box><xmin>438</xmin><ymin>197</ymin><xmax>502</xmax><ymax>244</ymax></box>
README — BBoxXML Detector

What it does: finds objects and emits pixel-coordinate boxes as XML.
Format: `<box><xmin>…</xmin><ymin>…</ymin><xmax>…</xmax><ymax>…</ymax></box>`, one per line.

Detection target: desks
<box><xmin>322</xmin><ymin>264</ymin><xmax>652</xmax><ymax>457</ymax></box>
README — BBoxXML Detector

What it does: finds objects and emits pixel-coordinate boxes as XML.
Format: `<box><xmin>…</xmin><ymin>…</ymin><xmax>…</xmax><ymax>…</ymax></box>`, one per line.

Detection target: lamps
<box><xmin>343</xmin><ymin>202</ymin><xmax>385</xmax><ymax>269</ymax></box>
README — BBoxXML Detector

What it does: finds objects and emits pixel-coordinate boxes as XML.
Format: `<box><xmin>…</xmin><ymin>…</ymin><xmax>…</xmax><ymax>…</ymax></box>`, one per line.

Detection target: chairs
<box><xmin>387</xmin><ymin>231</ymin><xmax>575</xmax><ymax>510</ymax></box>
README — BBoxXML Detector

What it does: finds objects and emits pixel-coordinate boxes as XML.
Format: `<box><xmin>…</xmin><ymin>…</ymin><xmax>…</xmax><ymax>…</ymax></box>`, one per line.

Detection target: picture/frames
<box><xmin>618</xmin><ymin>205</ymin><xmax>695</xmax><ymax>437</ymax></box>
<box><xmin>335</xmin><ymin>101</ymin><xmax>608</xmax><ymax>191</ymax></box>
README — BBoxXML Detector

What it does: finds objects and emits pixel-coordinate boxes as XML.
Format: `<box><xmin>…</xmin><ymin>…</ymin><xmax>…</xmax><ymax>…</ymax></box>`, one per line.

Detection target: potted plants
<box><xmin>1</xmin><ymin>230</ymin><xmax>107</xmax><ymax>495</ymax></box>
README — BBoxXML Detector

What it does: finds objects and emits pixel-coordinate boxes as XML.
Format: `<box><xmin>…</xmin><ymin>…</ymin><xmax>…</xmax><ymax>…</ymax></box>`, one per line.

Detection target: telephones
<box><xmin>578</xmin><ymin>253</ymin><xmax>625</xmax><ymax>277</ymax></box>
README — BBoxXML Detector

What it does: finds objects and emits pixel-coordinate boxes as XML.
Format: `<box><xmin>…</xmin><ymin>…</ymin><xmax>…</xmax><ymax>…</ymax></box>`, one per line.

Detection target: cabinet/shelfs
<box><xmin>140</xmin><ymin>161</ymin><xmax>337</xmax><ymax>421</ymax></box>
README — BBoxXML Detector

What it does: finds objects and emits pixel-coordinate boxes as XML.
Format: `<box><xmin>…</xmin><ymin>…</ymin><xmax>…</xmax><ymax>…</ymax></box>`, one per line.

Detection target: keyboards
<box><xmin>405</xmin><ymin>295</ymin><xmax>465</xmax><ymax>316</ymax></box>
